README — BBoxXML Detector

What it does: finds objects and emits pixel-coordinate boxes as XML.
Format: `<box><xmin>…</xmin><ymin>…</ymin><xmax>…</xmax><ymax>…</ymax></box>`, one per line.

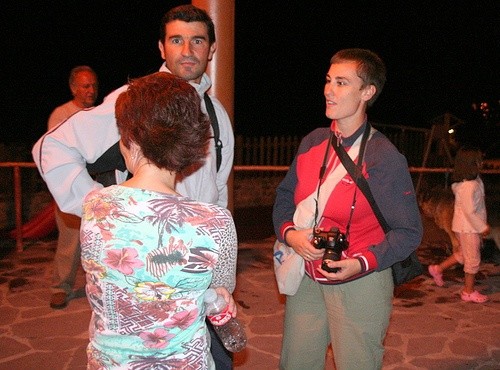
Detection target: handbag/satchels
<box><xmin>272</xmin><ymin>127</ymin><xmax>374</xmax><ymax>297</ymax></box>
<box><xmin>393</xmin><ymin>250</ymin><xmax>423</xmax><ymax>286</ymax></box>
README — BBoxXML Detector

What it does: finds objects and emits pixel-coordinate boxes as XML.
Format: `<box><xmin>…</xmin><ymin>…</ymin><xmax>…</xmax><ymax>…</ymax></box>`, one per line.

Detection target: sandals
<box><xmin>428</xmin><ymin>263</ymin><xmax>444</xmax><ymax>287</ymax></box>
<box><xmin>461</xmin><ymin>290</ymin><xmax>489</xmax><ymax>303</ymax></box>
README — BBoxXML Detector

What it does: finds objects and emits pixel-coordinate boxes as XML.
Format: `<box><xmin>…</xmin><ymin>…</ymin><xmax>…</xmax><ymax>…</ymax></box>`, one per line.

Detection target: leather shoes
<box><xmin>50</xmin><ymin>292</ymin><xmax>66</xmax><ymax>307</ymax></box>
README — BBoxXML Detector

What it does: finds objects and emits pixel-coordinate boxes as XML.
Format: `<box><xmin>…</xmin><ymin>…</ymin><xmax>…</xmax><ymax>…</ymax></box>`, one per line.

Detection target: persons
<box><xmin>32</xmin><ymin>5</ymin><xmax>236</xmax><ymax>370</ymax></box>
<box><xmin>427</xmin><ymin>145</ymin><xmax>490</xmax><ymax>302</ymax></box>
<box><xmin>48</xmin><ymin>66</ymin><xmax>97</xmax><ymax>306</ymax></box>
<box><xmin>80</xmin><ymin>70</ymin><xmax>236</xmax><ymax>370</ymax></box>
<box><xmin>272</xmin><ymin>48</ymin><xmax>423</xmax><ymax>370</ymax></box>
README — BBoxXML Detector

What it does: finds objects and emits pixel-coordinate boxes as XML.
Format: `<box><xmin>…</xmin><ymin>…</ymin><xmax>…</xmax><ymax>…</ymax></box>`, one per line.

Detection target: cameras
<box><xmin>311</xmin><ymin>227</ymin><xmax>349</xmax><ymax>272</ymax></box>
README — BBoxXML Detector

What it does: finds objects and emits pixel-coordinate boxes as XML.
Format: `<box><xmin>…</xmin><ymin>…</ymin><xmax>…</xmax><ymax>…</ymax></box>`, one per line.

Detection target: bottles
<box><xmin>203</xmin><ymin>288</ymin><xmax>247</xmax><ymax>353</ymax></box>
